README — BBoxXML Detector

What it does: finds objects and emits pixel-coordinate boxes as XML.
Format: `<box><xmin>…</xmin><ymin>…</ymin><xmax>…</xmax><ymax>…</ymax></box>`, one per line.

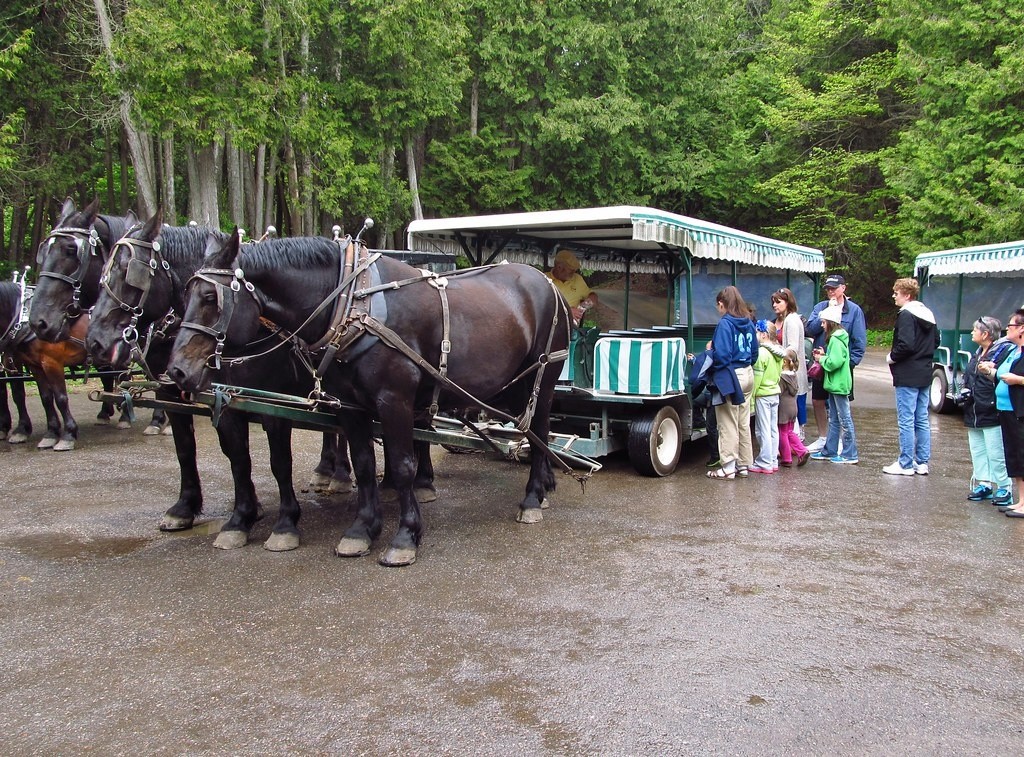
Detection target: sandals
<box><xmin>735</xmin><ymin>467</ymin><xmax>748</xmax><ymax>478</ymax></box>
<box><xmin>706</xmin><ymin>468</ymin><xmax>736</xmax><ymax>480</ymax></box>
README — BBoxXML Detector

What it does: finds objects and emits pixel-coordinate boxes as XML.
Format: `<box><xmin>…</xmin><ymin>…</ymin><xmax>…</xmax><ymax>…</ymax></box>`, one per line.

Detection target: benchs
<box><xmin>932</xmin><ymin>326</ymin><xmax>1007</xmax><ymax>372</ymax></box>
<box><xmin>597</xmin><ymin>320</ymin><xmax>721</xmax><ymax>384</ymax></box>
<box><xmin>571</xmin><ymin>301</ymin><xmax>597</xmax><ymax>386</ymax></box>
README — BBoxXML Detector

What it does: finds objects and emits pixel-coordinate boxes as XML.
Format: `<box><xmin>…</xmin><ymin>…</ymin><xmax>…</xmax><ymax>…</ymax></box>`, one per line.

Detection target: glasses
<box><xmin>825</xmin><ymin>287</ymin><xmax>835</xmax><ymax>291</ymax></box>
<box><xmin>893</xmin><ymin>292</ymin><xmax>898</xmax><ymax>296</ymax></box>
<box><xmin>777</xmin><ymin>288</ymin><xmax>788</xmax><ymax>301</ymax></box>
<box><xmin>1007</xmin><ymin>323</ymin><xmax>1021</xmax><ymax>328</ymax></box>
<box><xmin>714</xmin><ymin>299</ymin><xmax>723</xmax><ymax>309</ymax></box>
<box><xmin>978</xmin><ymin>316</ymin><xmax>989</xmax><ymax>329</ymax></box>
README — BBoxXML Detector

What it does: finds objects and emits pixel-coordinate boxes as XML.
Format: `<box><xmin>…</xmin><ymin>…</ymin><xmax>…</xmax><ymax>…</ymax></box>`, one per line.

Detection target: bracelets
<box><xmin>984</xmin><ymin>370</ymin><xmax>991</xmax><ymax>375</ymax></box>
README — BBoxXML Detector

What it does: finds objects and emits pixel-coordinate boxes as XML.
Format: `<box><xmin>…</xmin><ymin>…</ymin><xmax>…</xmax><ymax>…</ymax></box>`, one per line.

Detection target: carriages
<box><xmin>30</xmin><ymin>194</ymin><xmax>824</xmax><ymax>567</ymax></box>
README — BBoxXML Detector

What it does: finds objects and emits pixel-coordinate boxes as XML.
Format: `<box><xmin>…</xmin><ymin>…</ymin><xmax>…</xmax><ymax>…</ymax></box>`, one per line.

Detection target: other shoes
<box><xmin>998</xmin><ymin>506</ymin><xmax>1015</xmax><ymax>512</ymax></box>
<box><xmin>1005</xmin><ymin>510</ymin><xmax>1024</xmax><ymax>518</ymax></box>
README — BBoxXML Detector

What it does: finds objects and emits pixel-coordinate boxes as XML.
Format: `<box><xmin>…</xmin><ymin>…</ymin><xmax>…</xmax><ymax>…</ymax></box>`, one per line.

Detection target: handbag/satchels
<box><xmin>808</xmin><ymin>348</ymin><xmax>823</xmax><ymax>383</ymax></box>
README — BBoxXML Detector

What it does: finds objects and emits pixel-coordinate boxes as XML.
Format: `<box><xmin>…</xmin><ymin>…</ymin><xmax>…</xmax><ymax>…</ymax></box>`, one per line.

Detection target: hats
<box><xmin>820</xmin><ymin>301</ymin><xmax>843</xmax><ymax>324</ymax></box>
<box><xmin>555</xmin><ymin>250</ymin><xmax>582</xmax><ymax>269</ymax></box>
<box><xmin>822</xmin><ymin>275</ymin><xmax>845</xmax><ymax>288</ymax></box>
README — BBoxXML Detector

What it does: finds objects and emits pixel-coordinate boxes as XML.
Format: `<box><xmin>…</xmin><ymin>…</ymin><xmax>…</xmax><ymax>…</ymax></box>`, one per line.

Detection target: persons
<box><xmin>543</xmin><ymin>250</ymin><xmax>592</xmax><ymax>328</ymax></box>
<box><xmin>750</xmin><ymin>319</ymin><xmax>786</xmax><ymax>473</ymax></box>
<box><xmin>686</xmin><ymin>275</ymin><xmax>759</xmax><ymax>480</ymax></box>
<box><xmin>805</xmin><ymin>274</ymin><xmax>866</xmax><ymax>455</ymax></box>
<box><xmin>882</xmin><ymin>276</ymin><xmax>941</xmax><ymax>475</ymax></box>
<box><xmin>810</xmin><ymin>305</ymin><xmax>859</xmax><ymax>464</ymax></box>
<box><xmin>779</xmin><ymin>347</ymin><xmax>810</xmax><ymax>468</ymax></box>
<box><xmin>771</xmin><ymin>288</ymin><xmax>807</xmax><ymax>455</ymax></box>
<box><xmin>961</xmin><ymin>315</ymin><xmax>1014</xmax><ymax>505</ymax></box>
<box><xmin>978</xmin><ymin>308</ymin><xmax>1024</xmax><ymax>518</ymax></box>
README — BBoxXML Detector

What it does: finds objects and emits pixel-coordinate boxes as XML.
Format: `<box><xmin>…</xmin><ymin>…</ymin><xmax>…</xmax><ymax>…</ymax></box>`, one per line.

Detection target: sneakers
<box><xmin>838</xmin><ymin>439</ymin><xmax>843</xmax><ymax>455</ymax></box>
<box><xmin>778</xmin><ymin>460</ymin><xmax>792</xmax><ymax>467</ymax></box>
<box><xmin>810</xmin><ymin>452</ymin><xmax>838</xmax><ymax>460</ymax></box>
<box><xmin>773</xmin><ymin>460</ymin><xmax>778</xmax><ymax>471</ymax></box>
<box><xmin>798</xmin><ymin>451</ymin><xmax>810</xmax><ymax>466</ymax></box>
<box><xmin>830</xmin><ymin>455</ymin><xmax>858</xmax><ymax>464</ymax></box>
<box><xmin>748</xmin><ymin>462</ymin><xmax>773</xmax><ymax>474</ymax></box>
<box><xmin>991</xmin><ymin>487</ymin><xmax>1013</xmax><ymax>505</ymax></box>
<box><xmin>883</xmin><ymin>461</ymin><xmax>915</xmax><ymax>476</ymax></box>
<box><xmin>967</xmin><ymin>484</ymin><xmax>994</xmax><ymax>501</ymax></box>
<box><xmin>912</xmin><ymin>459</ymin><xmax>929</xmax><ymax>475</ymax></box>
<box><xmin>706</xmin><ymin>451</ymin><xmax>720</xmax><ymax>466</ymax></box>
<box><xmin>805</xmin><ymin>436</ymin><xmax>828</xmax><ymax>453</ymax></box>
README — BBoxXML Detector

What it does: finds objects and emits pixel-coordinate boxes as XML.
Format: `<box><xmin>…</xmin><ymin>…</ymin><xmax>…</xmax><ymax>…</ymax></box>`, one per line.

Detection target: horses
<box><xmin>0</xmin><ymin>280</ymin><xmax>175</xmax><ymax>451</ymax></box>
<box><xmin>167</xmin><ymin>226</ymin><xmax>575</xmax><ymax>565</ymax></box>
<box><xmin>29</xmin><ymin>196</ymin><xmax>354</xmax><ymax>532</ymax></box>
<box><xmin>84</xmin><ymin>204</ymin><xmax>438</xmax><ymax>551</ymax></box>
